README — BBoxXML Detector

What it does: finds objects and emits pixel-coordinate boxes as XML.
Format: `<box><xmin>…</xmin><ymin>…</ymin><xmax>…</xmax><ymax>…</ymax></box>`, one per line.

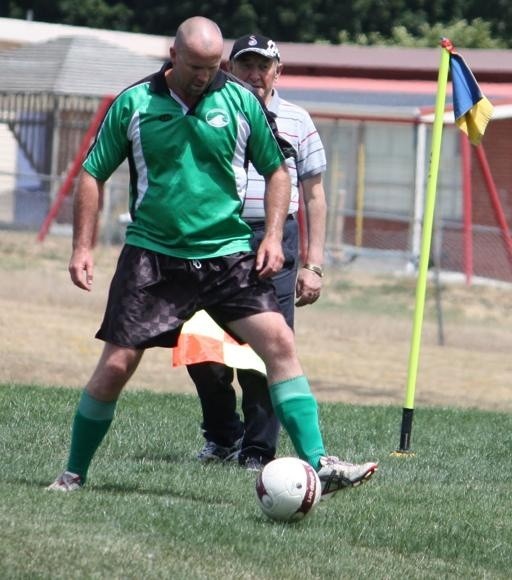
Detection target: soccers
<box><xmin>254</xmin><ymin>457</ymin><xmax>322</xmax><ymax>524</ymax></box>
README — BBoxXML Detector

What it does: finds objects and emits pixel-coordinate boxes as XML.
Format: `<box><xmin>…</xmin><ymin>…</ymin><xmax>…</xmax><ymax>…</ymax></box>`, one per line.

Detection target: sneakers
<box><xmin>238</xmin><ymin>446</ymin><xmax>270</xmax><ymax>471</ymax></box>
<box><xmin>317</xmin><ymin>455</ymin><xmax>379</xmax><ymax>496</ymax></box>
<box><xmin>44</xmin><ymin>470</ymin><xmax>82</xmax><ymax>491</ymax></box>
<box><xmin>196</xmin><ymin>433</ymin><xmax>245</xmax><ymax>465</ymax></box>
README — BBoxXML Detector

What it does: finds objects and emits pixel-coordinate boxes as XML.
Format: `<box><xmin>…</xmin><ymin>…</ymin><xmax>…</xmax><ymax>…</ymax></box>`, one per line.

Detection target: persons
<box><xmin>185</xmin><ymin>31</ymin><xmax>328</xmax><ymax>479</ymax></box>
<box><xmin>45</xmin><ymin>14</ymin><xmax>381</xmax><ymax>497</ymax></box>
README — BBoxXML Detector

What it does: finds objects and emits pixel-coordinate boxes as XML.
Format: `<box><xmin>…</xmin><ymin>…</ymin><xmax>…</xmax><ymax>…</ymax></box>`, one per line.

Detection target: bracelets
<box><xmin>301</xmin><ymin>263</ymin><xmax>324</xmax><ymax>278</ymax></box>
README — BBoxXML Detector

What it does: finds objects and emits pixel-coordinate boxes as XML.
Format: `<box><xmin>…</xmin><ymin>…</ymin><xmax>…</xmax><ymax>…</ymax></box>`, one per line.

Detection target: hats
<box><xmin>229</xmin><ymin>32</ymin><xmax>281</xmax><ymax>63</ymax></box>
